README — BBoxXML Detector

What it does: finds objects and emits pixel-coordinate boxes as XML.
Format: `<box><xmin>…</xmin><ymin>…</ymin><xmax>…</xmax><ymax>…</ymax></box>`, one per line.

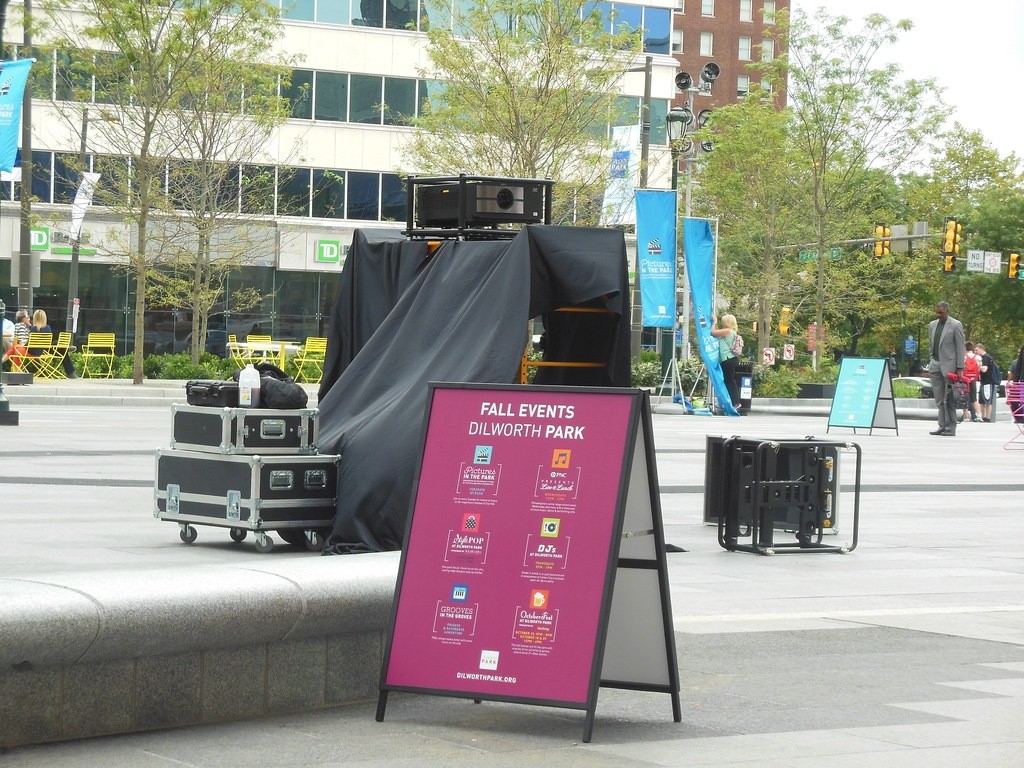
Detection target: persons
<box><xmin>962</xmin><ymin>341</ymin><xmax>1024</xmax><ymax>423</ymax></box>
<box><xmin>710</xmin><ymin>314</ymin><xmax>742</xmax><ymax>410</ymax></box>
<box><xmin>928</xmin><ymin>301</ymin><xmax>965</xmax><ymax>436</ymax></box>
<box><xmin>0</xmin><ymin>310</ymin><xmax>80</xmax><ymax>379</ymax></box>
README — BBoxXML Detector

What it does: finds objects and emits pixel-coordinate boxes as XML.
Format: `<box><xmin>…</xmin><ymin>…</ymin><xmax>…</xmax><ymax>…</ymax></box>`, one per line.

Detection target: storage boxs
<box><xmin>186</xmin><ymin>380</ymin><xmax>238</xmax><ymax>407</ymax></box>
<box><xmin>154</xmin><ymin>447</ymin><xmax>341</xmax><ymax>532</ymax></box>
<box><xmin>170</xmin><ymin>403</ymin><xmax>320</xmax><ymax>456</ymax></box>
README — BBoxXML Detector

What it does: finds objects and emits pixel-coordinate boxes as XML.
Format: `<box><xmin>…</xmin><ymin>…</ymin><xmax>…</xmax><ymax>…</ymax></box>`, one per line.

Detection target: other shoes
<box><xmin>983</xmin><ymin>418</ymin><xmax>990</xmax><ymax>423</ymax></box>
<box><xmin>68</xmin><ymin>373</ymin><xmax>82</xmax><ymax>379</ymax></box>
<box><xmin>972</xmin><ymin>417</ymin><xmax>979</xmax><ymax>422</ymax></box>
<box><xmin>734</xmin><ymin>404</ymin><xmax>741</xmax><ymax>409</ymax></box>
<box><xmin>962</xmin><ymin>415</ymin><xmax>970</xmax><ymax>421</ymax></box>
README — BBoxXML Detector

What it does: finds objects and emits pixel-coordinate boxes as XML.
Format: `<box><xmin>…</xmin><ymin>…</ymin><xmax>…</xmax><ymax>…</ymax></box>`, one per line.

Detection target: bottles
<box><xmin>0</xmin><ymin>299</ymin><xmax>5</xmax><ymax>317</ymax></box>
<box><xmin>238</xmin><ymin>364</ymin><xmax>261</xmax><ymax>408</ymax></box>
<box><xmin>699</xmin><ymin>315</ymin><xmax>706</xmax><ymax>327</ymax></box>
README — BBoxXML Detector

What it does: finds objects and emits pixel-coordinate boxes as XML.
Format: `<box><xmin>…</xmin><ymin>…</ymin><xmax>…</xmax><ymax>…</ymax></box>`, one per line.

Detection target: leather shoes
<box><xmin>943</xmin><ymin>431</ymin><xmax>956</xmax><ymax>436</ymax></box>
<box><xmin>929</xmin><ymin>431</ymin><xmax>941</xmax><ymax>435</ymax></box>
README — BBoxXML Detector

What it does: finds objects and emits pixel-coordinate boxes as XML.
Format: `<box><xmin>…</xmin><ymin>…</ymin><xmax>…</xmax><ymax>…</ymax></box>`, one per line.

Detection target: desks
<box><xmin>226</xmin><ymin>342</ymin><xmax>301</xmax><ymax>367</ymax></box>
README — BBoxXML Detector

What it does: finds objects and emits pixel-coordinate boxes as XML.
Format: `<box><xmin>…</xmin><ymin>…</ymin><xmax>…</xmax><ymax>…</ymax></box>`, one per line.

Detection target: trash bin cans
<box><xmin>735</xmin><ymin>361</ymin><xmax>757</xmax><ymax>412</ymax></box>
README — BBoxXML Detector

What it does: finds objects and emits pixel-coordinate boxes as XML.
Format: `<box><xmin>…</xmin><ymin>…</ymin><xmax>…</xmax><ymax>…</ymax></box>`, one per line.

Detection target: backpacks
<box><xmin>732</xmin><ymin>335</ymin><xmax>744</xmax><ymax>355</ymax></box>
<box><xmin>983</xmin><ymin>355</ymin><xmax>1001</xmax><ymax>385</ymax></box>
<box><xmin>963</xmin><ymin>354</ymin><xmax>980</xmax><ymax>381</ymax></box>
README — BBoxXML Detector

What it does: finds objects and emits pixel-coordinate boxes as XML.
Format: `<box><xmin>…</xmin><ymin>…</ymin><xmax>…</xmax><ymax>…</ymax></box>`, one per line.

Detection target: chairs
<box><xmin>1003</xmin><ymin>380</ymin><xmax>1024</xmax><ymax>450</ymax></box>
<box><xmin>229</xmin><ymin>334</ymin><xmax>328</xmax><ymax>384</ymax></box>
<box><xmin>8</xmin><ymin>331</ymin><xmax>117</xmax><ymax>379</ymax></box>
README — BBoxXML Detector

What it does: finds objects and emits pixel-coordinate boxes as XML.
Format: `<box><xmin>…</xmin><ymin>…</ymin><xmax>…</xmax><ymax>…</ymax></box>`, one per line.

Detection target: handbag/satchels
<box><xmin>948</xmin><ymin>383</ymin><xmax>969</xmax><ymax>409</ymax></box>
